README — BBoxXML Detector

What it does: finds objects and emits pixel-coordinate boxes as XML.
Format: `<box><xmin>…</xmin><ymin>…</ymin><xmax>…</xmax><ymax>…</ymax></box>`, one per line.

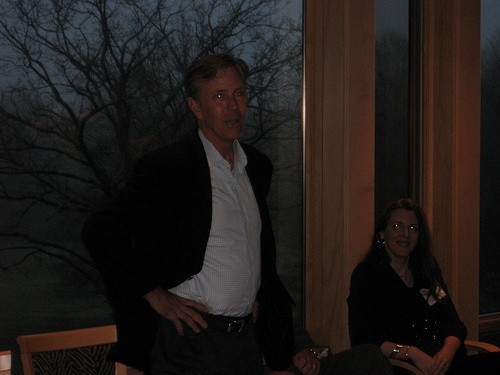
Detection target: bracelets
<box><xmin>391</xmin><ymin>344</ymin><xmax>410</xmax><ymax>360</ymax></box>
<box><xmin>303</xmin><ymin>346</ymin><xmax>320</xmax><ymax>357</ymax></box>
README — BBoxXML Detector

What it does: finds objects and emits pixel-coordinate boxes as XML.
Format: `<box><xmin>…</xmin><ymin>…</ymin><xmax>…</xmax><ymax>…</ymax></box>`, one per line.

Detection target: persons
<box><xmin>350</xmin><ymin>200</ymin><xmax>467</xmax><ymax>375</ymax></box>
<box><xmin>80</xmin><ymin>53</ymin><xmax>320</xmax><ymax>375</ymax></box>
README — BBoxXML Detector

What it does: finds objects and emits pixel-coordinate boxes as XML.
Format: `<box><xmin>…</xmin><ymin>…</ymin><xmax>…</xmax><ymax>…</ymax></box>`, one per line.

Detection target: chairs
<box><xmin>16</xmin><ymin>325</ymin><xmax>127</xmax><ymax>375</ymax></box>
<box><xmin>387</xmin><ymin>340</ymin><xmax>500</xmax><ymax>375</ymax></box>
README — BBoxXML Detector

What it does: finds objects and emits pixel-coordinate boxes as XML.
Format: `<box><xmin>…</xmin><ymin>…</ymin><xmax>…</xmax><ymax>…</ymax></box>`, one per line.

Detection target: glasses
<box><xmin>388</xmin><ymin>222</ymin><xmax>421</xmax><ymax>232</ymax></box>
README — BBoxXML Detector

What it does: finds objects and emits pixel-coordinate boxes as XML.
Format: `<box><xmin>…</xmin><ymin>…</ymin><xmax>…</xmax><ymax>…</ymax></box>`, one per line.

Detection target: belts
<box><xmin>203</xmin><ymin>318</ymin><xmax>254</xmax><ymax>334</ymax></box>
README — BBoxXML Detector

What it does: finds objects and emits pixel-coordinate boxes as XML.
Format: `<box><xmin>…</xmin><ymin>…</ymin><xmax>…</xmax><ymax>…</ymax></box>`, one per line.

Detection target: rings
<box><xmin>435</xmin><ymin>370</ymin><xmax>440</xmax><ymax>375</ymax></box>
<box><xmin>312</xmin><ymin>362</ymin><xmax>319</xmax><ymax>368</ymax></box>
